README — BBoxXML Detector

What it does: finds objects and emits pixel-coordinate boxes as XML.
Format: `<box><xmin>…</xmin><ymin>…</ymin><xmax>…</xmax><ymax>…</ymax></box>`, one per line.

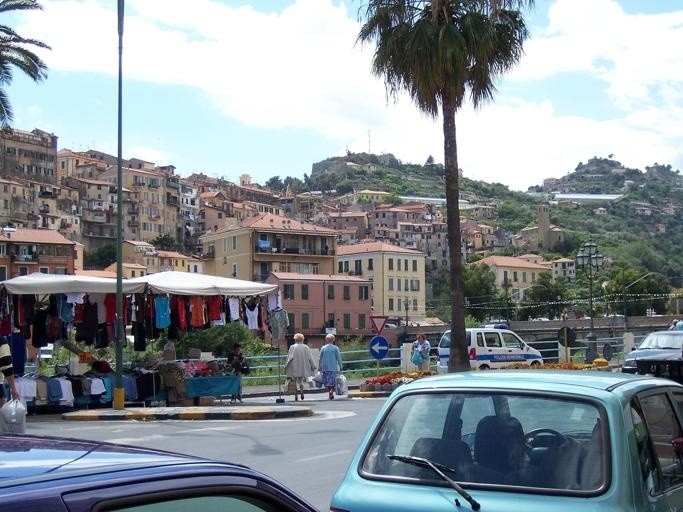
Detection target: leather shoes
<box><xmin>295</xmin><ymin>391</ymin><xmax>334</xmax><ymax>401</ymax></box>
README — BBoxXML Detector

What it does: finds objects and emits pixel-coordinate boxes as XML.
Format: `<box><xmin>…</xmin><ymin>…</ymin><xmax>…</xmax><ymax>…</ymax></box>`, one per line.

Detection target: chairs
<box><xmin>405</xmin><ymin>415</ymin><xmax>585</xmax><ymax>487</ymax></box>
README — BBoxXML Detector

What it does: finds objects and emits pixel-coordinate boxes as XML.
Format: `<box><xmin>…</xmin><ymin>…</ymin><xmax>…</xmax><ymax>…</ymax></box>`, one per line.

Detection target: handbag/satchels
<box><xmin>411</xmin><ymin>351</ymin><xmax>425</xmax><ymax>365</ymax></box>
<box><xmin>282</xmin><ymin>379</ymin><xmax>295</xmax><ymax>395</ymax></box>
<box><xmin>334</xmin><ymin>375</ymin><xmax>346</xmax><ymax>395</ymax></box>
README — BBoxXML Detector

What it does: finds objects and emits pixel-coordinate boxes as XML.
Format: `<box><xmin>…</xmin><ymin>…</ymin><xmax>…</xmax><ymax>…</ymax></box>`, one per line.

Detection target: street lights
<box><xmin>556</xmin><ymin>294</ymin><xmax>561</xmax><ymax>320</ymax></box>
<box><xmin>576</xmin><ymin>236</ymin><xmax>604</xmax><ymax>365</ymax></box>
<box><xmin>623</xmin><ymin>271</ymin><xmax>663</xmax><ymax>317</ymax></box>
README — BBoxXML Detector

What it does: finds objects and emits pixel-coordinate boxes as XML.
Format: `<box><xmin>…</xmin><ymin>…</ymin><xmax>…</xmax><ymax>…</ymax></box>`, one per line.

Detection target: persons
<box><xmin>284</xmin><ymin>332</ymin><xmax>316</xmax><ymax>401</ymax></box>
<box><xmin>317</xmin><ymin>333</ymin><xmax>342</xmax><ymax>399</ymax></box>
<box><xmin>667</xmin><ymin>318</ymin><xmax>677</xmax><ymax>330</ymax></box>
<box><xmin>0</xmin><ymin>334</ymin><xmax>19</xmax><ymax>407</ymax></box>
<box><xmin>410</xmin><ymin>333</ymin><xmax>430</xmax><ymax>372</ymax></box>
<box><xmin>227</xmin><ymin>343</ymin><xmax>244</xmax><ymax>404</ymax></box>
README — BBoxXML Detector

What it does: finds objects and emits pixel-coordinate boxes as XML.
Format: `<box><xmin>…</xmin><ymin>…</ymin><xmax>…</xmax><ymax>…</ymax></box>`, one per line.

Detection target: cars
<box><xmin>0</xmin><ymin>433</ymin><xmax>320</xmax><ymax>512</ymax></box>
<box><xmin>385</xmin><ymin>323</ymin><xmax>396</xmax><ymax>328</ymax></box>
<box><xmin>533</xmin><ymin>317</ymin><xmax>550</xmax><ymax>321</ymax></box>
<box><xmin>329</xmin><ymin>371</ymin><xmax>682</xmax><ymax>512</ymax></box>
<box><xmin>35</xmin><ymin>343</ymin><xmax>53</xmax><ymax>364</ymax></box>
<box><xmin>621</xmin><ymin>330</ymin><xmax>683</xmax><ymax>379</ymax></box>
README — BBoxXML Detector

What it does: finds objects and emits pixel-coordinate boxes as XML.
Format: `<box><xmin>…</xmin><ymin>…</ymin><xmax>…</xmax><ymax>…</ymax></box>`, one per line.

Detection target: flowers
<box><xmin>509</xmin><ymin>356</ymin><xmax>609</xmax><ymax>370</ymax></box>
<box><xmin>363</xmin><ymin>369</ymin><xmax>437</xmax><ymax>383</ymax></box>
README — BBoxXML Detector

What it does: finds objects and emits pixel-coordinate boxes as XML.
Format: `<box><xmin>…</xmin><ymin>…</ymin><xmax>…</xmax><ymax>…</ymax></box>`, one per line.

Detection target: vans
<box><xmin>436</xmin><ymin>322</ymin><xmax>545</xmax><ymax>372</ymax></box>
